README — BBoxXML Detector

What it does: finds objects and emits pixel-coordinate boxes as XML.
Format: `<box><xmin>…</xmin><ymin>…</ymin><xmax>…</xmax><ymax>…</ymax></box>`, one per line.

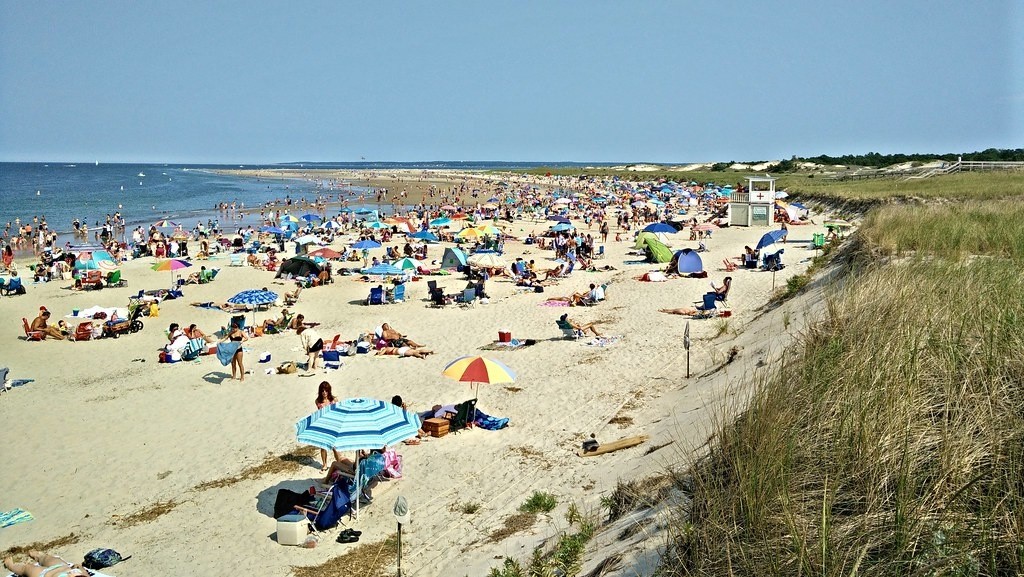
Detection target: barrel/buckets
<box><xmin>276</xmin><ymin>514</ymin><xmax>307</xmax><ymax>546</ymax></box>
<box><xmin>259</xmin><ymin>352</ymin><xmax>272</xmax><ymax>362</ymax></box>
<box><xmin>72</xmin><ymin>309</ymin><xmax>79</xmax><ymax>316</ymax></box>
<box><xmin>498</xmin><ymin>331</ymin><xmax>511</xmax><ymax>342</ymax></box>
<box><xmin>357</xmin><ymin>341</ymin><xmax>370</xmax><ymax>353</ymax></box>
<box><xmin>720</xmin><ymin>308</ymin><xmax>731</xmax><ymax>317</ymax></box>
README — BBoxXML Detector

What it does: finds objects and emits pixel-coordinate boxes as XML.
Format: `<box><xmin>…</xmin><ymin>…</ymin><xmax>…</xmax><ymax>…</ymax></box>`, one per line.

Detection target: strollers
<box><xmin>125</xmin><ymin>289</ymin><xmax>147</xmax><ymax>332</ymax></box>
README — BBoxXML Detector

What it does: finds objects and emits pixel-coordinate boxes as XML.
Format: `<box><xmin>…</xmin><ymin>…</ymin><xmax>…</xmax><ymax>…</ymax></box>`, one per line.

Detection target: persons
<box><xmin>314</xmin><ymin>381</ymin><xmax>345</xmax><ymax>474</ymax></box>
<box><xmin>313</xmin><ymin>445</ymin><xmax>387</xmax><ymax>485</ymax></box>
<box><xmin>389</xmin><ymin>395</ymin><xmax>432</xmax><ymax>439</ymax></box>
<box><xmin>1</xmin><ymin>169</ymin><xmax>839</xmax><ymax>382</ymax></box>
<box><xmin>431</xmin><ymin>404</ymin><xmax>509</xmax><ymax>430</ymax></box>
<box><xmin>3</xmin><ymin>549</ymin><xmax>91</xmax><ymax>577</ymax></box>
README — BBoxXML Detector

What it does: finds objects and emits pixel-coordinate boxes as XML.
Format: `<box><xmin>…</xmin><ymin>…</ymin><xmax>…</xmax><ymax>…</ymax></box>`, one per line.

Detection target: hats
<box><xmin>297</xmin><ymin>327</ymin><xmax>307</xmax><ymax>335</ymax></box>
<box><xmin>281</xmin><ymin>309</ymin><xmax>288</xmax><ymax>312</ymax></box>
<box><xmin>560</xmin><ymin>314</ymin><xmax>567</xmax><ymax>320</ymax></box>
<box><xmin>172</xmin><ymin>330</ymin><xmax>181</xmax><ymax>338</ymax></box>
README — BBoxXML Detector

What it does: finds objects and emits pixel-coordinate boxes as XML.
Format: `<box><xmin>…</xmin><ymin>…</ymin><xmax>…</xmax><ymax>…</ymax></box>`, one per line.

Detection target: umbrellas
<box><xmin>441</xmin><ymin>356</ymin><xmax>516</xmax><ymax>425</ymax></box>
<box><xmin>824</xmin><ymin>218</ymin><xmax>852</xmax><ymax>239</ymax></box>
<box><xmin>296</xmin><ymin>397</ymin><xmax>422</xmax><ymax>523</ymax></box>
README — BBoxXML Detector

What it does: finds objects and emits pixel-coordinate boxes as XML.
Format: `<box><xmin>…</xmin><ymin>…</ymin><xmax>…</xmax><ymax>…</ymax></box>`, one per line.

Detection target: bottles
<box><xmin>307</xmin><ymin>533</ymin><xmax>315</xmax><ymax>547</ymax></box>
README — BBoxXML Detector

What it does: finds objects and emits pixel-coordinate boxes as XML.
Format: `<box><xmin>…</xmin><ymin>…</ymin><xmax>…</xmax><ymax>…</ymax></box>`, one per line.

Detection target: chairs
<box><xmin>579</xmin><ymin>284</ymin><xmax>605</xmax><ymax>308</ymax></box>
<box><xmin>172</xmin><ymin>274</ymin><xmax>182</xmax><ymax>292</ymax></box>
<box><xmin>283</xmin><ymin>285</ymin><xmax>307</xmax><ymax>307</ymax></box>
<box><xmin>23</xmin><ymin>318</ymin><xmax>44</xmax><ymax>342</ymax></box>
<box><xmin>128</xmin><ymin>289</ymin><xmax>145</xmax><ymax>307</ymax></box>
<box><xmin>106</xmin><ymin>271</ymin><xmax>121</xmax><ymax>287</ymax></box>
<box><xmin>692</xmin><ymin>280</ymin><xmax>732</xmax><ymax>319</ymax></box>
<box><xmin>556</xmin><ymin>320</ymin><xmax>587</xmax><ymax>342</ymax></box>
<box><xmin>232</xmin><ymin>237</ymin><xmax>243</xmax><ymax>250</ymax></box>
<box><xmin>264</xmin><ymin>315</ymin><xmax>295</xmax><ymax>335</ymax></box>
<box><xmin>322</xmin><ymin>350</ymin><xmax>346</xmax><ymax>373</ymax></box>
<box><xmin>427</xmin><ymin>280</ymin><xmax>446</xmax><ymax>301</ymax></box>
<box><xmin>320</xmin><ymin>471</ymin><xmax>373</xmax><ymax>519</ymax></box>
<box><xmin>441</xmin><ymin>398</ymin><xmax>478</xmax><ymax>435</ymax></box>
<box><xmin>451</xmin><ymin>288</ymin><xmax>477</xmax><ymax>310</ymax></box>
<box><xmin>367</xmin><ymin>284</ymin><xmax>407</xmax><ymax>305</ymax></box>
<box><xmin>230</xmin><ymin>254</ymin><xmax>242</xmax><ymax>267</ymax></box>
<box><xmin>3</xmin><ymin>277</ymin><xmax>23</xmax><ymax>296</ymax></box>
<box><xmin>336</xmin><ymin>451</ymin><xmax>386</xmax><ymax>500</ymax></box>
<box><xmin>88</xmin><ymin>270</ymin><xmax>101</xmax><ymax>287</ymax></box>
<box><xmin>157</xmin><ymin>335</ymin><xmax>206</xmax><ymax>363</ymax></box>
<box><xmin>293</xmin><ymin>482</ymin><xmax>349</xmax><ymax>539</ymax></box>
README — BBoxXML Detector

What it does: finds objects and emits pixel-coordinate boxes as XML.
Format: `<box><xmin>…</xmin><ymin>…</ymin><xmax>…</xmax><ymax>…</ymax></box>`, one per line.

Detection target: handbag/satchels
<box><xmin>277</xmin><ymin>361</ymin><xmax>296</xmax><ymax>374</ymax></box>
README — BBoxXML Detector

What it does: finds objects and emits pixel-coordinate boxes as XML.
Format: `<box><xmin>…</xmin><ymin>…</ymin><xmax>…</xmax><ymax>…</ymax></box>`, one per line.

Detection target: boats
<box><xmin>138</xmin><ymin>173</ymin><xmax>145</xmax><ymax>177</ymax></box>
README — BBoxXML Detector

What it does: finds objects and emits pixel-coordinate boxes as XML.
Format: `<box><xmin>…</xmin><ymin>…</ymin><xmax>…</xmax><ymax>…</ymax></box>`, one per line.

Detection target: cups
<box><xmin>249</xmin><ymin>369</ymin><xmax>253</xmax><ymax>375</ymax></box>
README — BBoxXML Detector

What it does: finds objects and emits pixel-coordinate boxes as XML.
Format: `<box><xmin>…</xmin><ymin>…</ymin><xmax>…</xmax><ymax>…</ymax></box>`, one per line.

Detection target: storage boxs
<box><xmin>415</xmin><ymin>254</ymin><xmax>423</xmax><ymax>259</ymax></box>
<box><xmin>423</xmin><ymin>418</ymin><xmax>450</xmax><ymax>438</ymax></box>
<box><xmin>498</xmin><ymin>329</ymin><xmax>512</xmax><ymax>343</ymax></box>
<box><xmin>261</xmin><ymin>351</ymin><xmax>271</xmax><ymax>362</ymax></box>
<box><xmin>724</xmin><ymin>309</ymin><xmax>731</xmax><ymax>317</ymax></box>
<box><xmin>276</xmin><ymin>515</ymin><xmax>308</xmax><ymax>546</ymax></box>
<box><xmin>208</xmin><ymin>344</ymin><xmax>217</xmax><ymax>354</ymax></box>
<box><xmin>412</xmin><ymin>276</ymin><xmax>419</xmax><ymax>281</ymax></box>
<box><xmin>73</xmin><ymin>308</ymin><xmax>79</xmax><ymax>316</ymax></box>
<box><xmin>358</xmin><ymin>342</ymin><xmax>370</xmax><ymax>354</ymax></box>
<box><xmin>122</xmin><ymin>257</ymin><xmax>127</xmax><ymax>261</ymax></box>
<box><xmin>247</xmin><ymin>248</ymin><xmax>256</xmax><ymax>254</ymax></box>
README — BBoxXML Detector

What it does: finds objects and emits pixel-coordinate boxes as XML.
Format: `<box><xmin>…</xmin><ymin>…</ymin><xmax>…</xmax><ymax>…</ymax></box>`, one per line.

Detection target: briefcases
<box><xmin>259</xmin><ymin>352</ymin><xmax>271</xmax><ymax>362</ymax></box>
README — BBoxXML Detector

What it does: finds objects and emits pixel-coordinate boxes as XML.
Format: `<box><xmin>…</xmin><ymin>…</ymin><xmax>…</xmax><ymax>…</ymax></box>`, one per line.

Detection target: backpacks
<box><xmin>83</xmin><ymin>547</ymin><xmax>122</xmax><ymax>570</ymax></box>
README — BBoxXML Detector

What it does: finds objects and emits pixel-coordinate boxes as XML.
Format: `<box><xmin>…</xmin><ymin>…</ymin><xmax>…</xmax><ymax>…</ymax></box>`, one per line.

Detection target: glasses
<box><xmin>323</xmin><ymin>390</ymin><xmax>328</xmax><ymax>392</ymax></box>
<box><xmin>174</xmin><ymin>328</ymin><xmax>178</xmax><ymax>331</ymax></box>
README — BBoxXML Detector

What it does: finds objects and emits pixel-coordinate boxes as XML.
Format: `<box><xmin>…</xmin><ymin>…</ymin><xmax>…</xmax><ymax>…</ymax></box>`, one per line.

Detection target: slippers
<box><xmin>336</xmin><ymin>535</ymin><xmax>359</xmax><ymax>542</ymax></box>
<box><xmin>406</xmin><ymin>440</ymin><xmax>420</xmax><ymax>444</ymax></box>
<box><xmin>340</xmin><ymin>529</ymin><xmax>362</xmax><ymax>536</ymax></box>
